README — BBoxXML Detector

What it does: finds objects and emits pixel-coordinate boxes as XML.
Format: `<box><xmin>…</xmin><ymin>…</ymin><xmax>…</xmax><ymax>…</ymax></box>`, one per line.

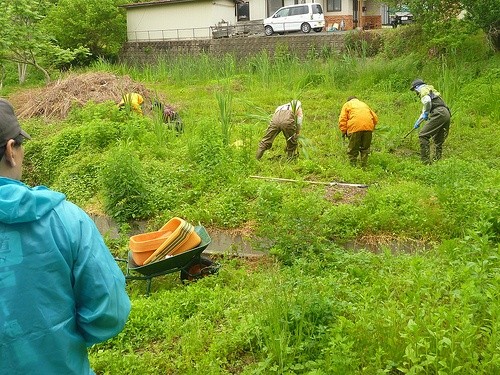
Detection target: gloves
<box><xmin>414</xmin><ymin>120</ymin><xmax>420</xmax><ymax>128</ymax></box>
<box><xmin>422</xmin><ymin>112</ymin><xmax>427</xmax><ymax>119</ymax></box>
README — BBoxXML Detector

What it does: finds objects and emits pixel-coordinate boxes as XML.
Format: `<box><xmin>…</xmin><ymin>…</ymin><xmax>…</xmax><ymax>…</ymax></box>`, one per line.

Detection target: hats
<box><xmin>0</xmin><ymin>99</ymin><xmax>31</xmax><ymax>147</ymax></box>
<box><xmin>410</xmin><ymin>79</ymin><xmax>425</xmax><ymax>91</ymax></box>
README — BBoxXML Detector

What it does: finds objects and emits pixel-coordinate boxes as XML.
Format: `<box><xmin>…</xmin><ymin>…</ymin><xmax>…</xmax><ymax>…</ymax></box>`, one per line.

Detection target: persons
<box><xmin>410</xmin><ymin>79</ymin><xmax>452</xmax><ymax>165</ymax></box>
<box><xmin>256</xmin><ymin>100</ymin><xmax>303</xmax><ymax>161</ymax></box>
<box><xmin>339</xmin><ymin>96</ymin><xmax>379</xmax><ymax>167</ymax></box>
<box><xmin>0</xmin><ymin>98</ymin><xmax>131</xmax><ymax>375</ymax></box>
<box><xmin>117</xmin><ymin>93</ymin><xmax>147</xmax><ymax>117</ymax></box>
<box><xmin>152</xmin><ymin>97</ymin><xmax>183</xmax><ymax>132</ymax></box>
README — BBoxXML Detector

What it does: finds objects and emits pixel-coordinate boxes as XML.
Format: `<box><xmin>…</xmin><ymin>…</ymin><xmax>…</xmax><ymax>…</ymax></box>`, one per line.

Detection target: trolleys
<box><xmin>115</xmin><ymin>225</ymin><xmax>219</xmax><ymax>297</ymax></box>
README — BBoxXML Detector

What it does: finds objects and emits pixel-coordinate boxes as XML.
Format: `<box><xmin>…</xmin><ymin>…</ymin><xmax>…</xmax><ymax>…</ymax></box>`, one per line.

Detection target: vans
<box><xmin>262</xmin><ymin>3</ymin><xmax>325</xmax><ymax>36</ymax></box>
